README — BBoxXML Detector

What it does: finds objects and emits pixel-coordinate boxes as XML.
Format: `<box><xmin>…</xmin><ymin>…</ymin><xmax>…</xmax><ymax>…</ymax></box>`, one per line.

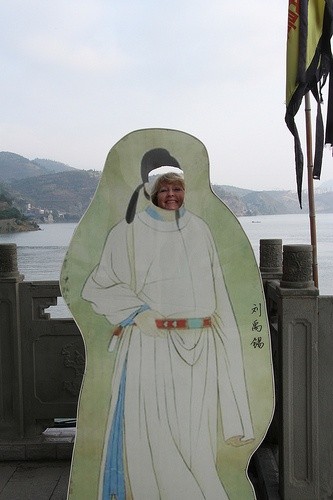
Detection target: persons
<box><xmin>150</xmin><ymin>171</ymin><xmax>186</xmax><ymax>210</ymax></box>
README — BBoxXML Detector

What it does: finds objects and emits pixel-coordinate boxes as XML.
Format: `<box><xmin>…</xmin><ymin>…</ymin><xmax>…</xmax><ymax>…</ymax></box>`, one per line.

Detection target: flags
<box><xmin>285</xmin><ymin>0</ymin><xmax>333</xmax><ymax>210</ymax></box>
